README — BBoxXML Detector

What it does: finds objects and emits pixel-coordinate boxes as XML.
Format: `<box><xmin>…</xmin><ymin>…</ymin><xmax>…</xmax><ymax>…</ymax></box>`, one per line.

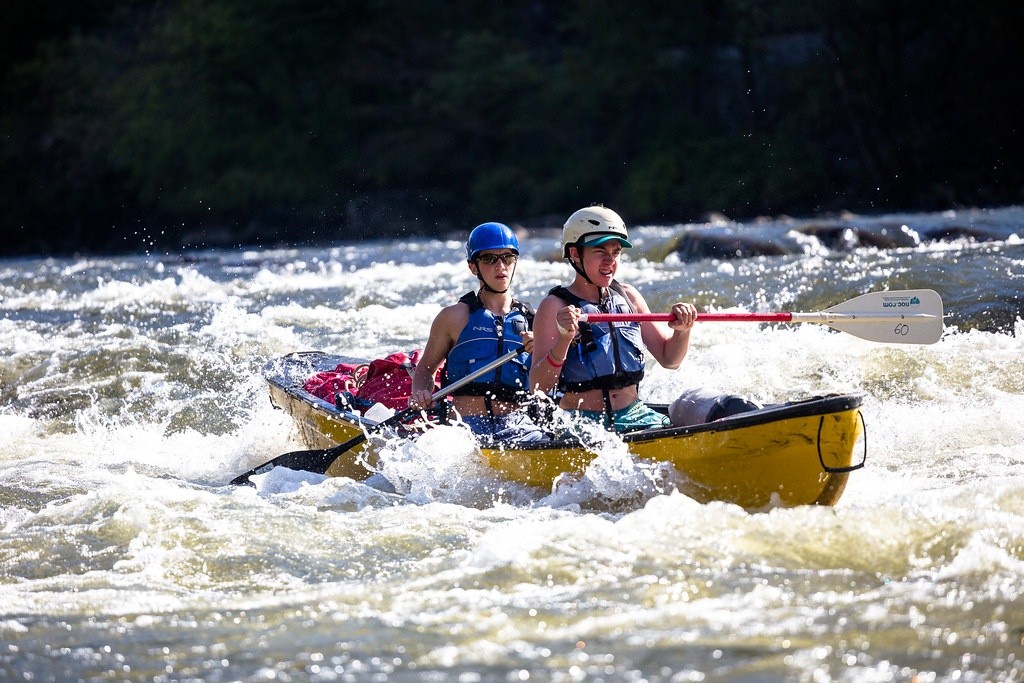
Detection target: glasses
<box><xmin>474</xmin><ymin>252</ymin><xmax>517</xmax><ymax>266</ymax></box>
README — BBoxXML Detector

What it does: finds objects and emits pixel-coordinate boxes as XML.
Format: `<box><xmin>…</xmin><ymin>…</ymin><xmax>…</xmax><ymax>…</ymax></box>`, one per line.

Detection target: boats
<box><xmin>259</xmin><ymin>348</ymin><xmax>864</xmax><ymax>520</ymax></box>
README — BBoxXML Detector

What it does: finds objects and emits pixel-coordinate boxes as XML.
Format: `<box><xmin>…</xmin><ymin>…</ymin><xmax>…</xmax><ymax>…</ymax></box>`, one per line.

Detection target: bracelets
<box><xmin>546</xmin><ymin>349</ymin><xmax>565</xmax><ymax>368</ymax></box>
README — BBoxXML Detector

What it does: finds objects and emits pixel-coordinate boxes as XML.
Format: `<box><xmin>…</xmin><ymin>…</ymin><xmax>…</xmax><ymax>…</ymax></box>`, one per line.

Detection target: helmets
<box><xmin>464</xmin><ymin>222</ymin><xmax>519</xmax><ymax>262</ymax></box>
<box><xmin>561</xmin><ymin>206</ymin><xmax>629</xmax><ymax>259</ymax></box>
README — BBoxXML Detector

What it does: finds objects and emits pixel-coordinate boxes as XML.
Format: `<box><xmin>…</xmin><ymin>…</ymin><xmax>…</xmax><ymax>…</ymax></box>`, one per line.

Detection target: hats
<box><xmin>581</xmin><ymin>233</ymin><xmax>633</xmax><ymax>249</ymax></box>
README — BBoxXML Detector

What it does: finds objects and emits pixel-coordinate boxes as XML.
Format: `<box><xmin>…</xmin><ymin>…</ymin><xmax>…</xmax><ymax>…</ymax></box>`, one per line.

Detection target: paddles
<box><xmin>579</xmin><ymin>288</ymin><xmax>945</xmax><ymax>345</ymax></box>
<box><xmin>224</xmin><ymin>342</ymin><xmax>528</xmax><ymax>488</ymax></box>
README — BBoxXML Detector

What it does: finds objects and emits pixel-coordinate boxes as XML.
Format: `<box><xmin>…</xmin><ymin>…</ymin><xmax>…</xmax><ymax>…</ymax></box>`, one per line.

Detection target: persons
<box><xmin>408</xmin><ymin>222</ymin><xmax>552</xmax><ymax>443</ymax></box>
<box><xmin>530</xmin><ymin>205</ymin><xmax>698</xmax><ymax>441</ymax></box>
